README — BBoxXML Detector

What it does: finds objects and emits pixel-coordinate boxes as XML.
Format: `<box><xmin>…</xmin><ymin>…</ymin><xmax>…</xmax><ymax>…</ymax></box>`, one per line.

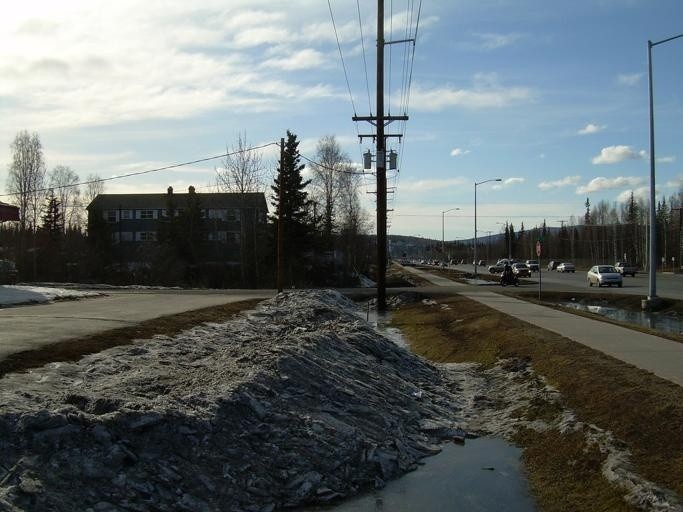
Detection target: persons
<box><xmin>503</xmin><ymin>264</ymin><xmax>512</xmax><ymax>281</ymax></box>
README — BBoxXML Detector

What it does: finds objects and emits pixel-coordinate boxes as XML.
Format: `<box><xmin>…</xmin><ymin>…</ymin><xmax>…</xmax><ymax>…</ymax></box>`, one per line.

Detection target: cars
<box><xmin>587</xmin><ymin>265</ymin><xmax>622</xmax><ymax>287</ymax></box>
<box><xmin>548</xmin><ymin>261</ymin><xmax>575</xmax><ymax>273</ymax></box>
<box><xmin>421</xmin><ymin>259</ymin><xmax>467</xmax><ymax>267</ymax></box>
<box><xmin>473</xmin><ymin>260</ymin><xmax>485</xmax><ymax>267</ymax></box>
<box><xmin>488</xmin><ymin>259</ymin><xmax>539</xmax><ymax>278</ymax></box>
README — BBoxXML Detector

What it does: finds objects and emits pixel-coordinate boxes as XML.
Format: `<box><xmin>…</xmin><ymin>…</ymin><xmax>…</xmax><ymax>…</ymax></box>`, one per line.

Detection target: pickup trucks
<box><xmin>614</xmin><ymin>262</ymin><xmax>638</xmax><ymax>277</ymax></box>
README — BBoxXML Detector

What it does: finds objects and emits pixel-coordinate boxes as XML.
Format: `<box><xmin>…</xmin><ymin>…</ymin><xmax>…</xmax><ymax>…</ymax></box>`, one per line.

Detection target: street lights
<box><xmin>442</xmin><ymin>208</ymin><xmax>460</xmax><ymax>272</ymax></box>
<box><xmin>475</xmin><ymin>179</ymin><xmax>501</xmax><ymax>273</ymax></box>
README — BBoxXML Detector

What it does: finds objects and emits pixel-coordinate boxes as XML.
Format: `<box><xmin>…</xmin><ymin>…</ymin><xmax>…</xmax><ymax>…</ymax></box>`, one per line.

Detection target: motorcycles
<box><xmin>500</xmin><ymin>271</ymin><xmax>521</xmax><ymax>286</ymax></box>
